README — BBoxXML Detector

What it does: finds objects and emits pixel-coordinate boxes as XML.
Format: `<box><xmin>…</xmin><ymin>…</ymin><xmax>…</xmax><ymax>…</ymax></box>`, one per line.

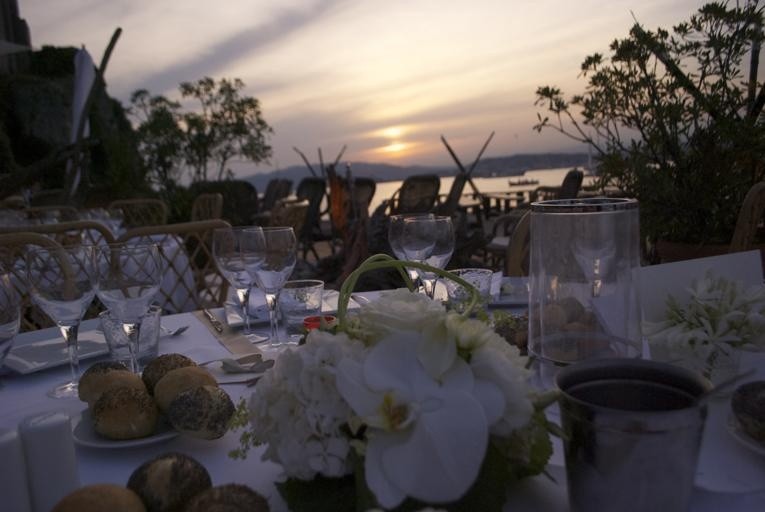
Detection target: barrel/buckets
<box><xmin>558</xmin><ymin>358</ymin><xmax>714</xmax><ymax>512</ymax></box>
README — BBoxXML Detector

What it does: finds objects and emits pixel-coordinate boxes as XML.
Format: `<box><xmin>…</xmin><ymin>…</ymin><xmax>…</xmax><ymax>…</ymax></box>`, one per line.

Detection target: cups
<box><xmin>445</xmin><ymin>266</ymin><xmax>493</xmax><ymax>320</ymax></box>
<box><xmin>528</xmin><ymin>192</ymin><xmax>645</xmax><ymax>374</ymax></box>
<box><xmin>305</xmin><ymin>312</ymin><xmax>334</xmax><ymax>331</ymax></box>
<box><xmin>277</xmin><ymin>278</ymin><xmax>325</xmax><ymax>339</ymax></box>
<box><xmin>97</xmin><ymin>303</ymin><xmax>159</xmax><ymax>372</ymax></box>
<box><xmin>554</xmin><ymin>358</ymin><xmax>714</xmax><ymax>512</ymax></box>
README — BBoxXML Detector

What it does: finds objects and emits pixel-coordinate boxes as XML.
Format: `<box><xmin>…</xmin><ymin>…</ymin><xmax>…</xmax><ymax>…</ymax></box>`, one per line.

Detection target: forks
<box><xmin>160</xmin><ymin>323</ymin><xmax>189</xmax><ymax>340</ymax></box>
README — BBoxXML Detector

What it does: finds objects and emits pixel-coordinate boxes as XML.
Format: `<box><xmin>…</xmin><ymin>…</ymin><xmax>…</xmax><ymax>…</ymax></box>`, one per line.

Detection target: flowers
<box><xmin>224</xmin><ymin>254</ymin><xmax>571</xmax><ymax>511</ymax></box>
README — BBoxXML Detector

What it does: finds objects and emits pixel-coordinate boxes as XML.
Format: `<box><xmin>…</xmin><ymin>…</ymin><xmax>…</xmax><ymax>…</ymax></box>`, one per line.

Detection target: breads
<box><xmin>49</xmin><ymin>452</ymin><xmax>272</xmax><ymax>512</ymax></box>
<box><xmin>78</xmin><ymin>353</ymin><xmax>237</xmax><ymax>440</ymax></box>
<box><xmin>514</xmin><ymin>296</ymin><xmax>600</xmax><ymax>361</ymax></box>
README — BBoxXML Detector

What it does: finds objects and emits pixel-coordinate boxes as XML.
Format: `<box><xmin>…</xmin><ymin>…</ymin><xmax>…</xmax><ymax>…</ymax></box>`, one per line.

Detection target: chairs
<box><xmin>1</xmin><ymin>169</ymin><xmax>765</xmax><ymax>337</ymax></box>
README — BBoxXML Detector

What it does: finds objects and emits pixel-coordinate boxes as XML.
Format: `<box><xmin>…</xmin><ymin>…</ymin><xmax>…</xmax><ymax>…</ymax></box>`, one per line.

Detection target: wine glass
<box><xmin>387</xmin><ymin>209</ymin><xmax>455</xmax><ymax>303</ymax></box>
<box><xmin>212</xmin><ymin>221</ymin><xmax>306</xmax><ymax>360</ymax></box>
<box><xmin>23</xmin><ymin>237</ymin><xmax>165</xmax><ymax>404</ymax></box>
<box><xmin>0</xmin><ymin>265</ymin><xmax>22</xmax><ymax>364</ymax></box>
<box><xmin>0</xmin><ymin>207</ymin><xmax>125</xmax><ymax>242</ymax></box>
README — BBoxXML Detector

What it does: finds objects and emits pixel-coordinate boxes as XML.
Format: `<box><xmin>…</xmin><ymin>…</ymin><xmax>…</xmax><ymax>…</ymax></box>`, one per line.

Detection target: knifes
<box><xmin>204</xmin><ymin>306</ymin><xmax>223</xmax><ymax>334</ymax></box>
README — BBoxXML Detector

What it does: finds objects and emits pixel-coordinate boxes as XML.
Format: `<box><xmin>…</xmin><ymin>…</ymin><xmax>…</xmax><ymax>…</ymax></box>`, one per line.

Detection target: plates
<box><xmin>220</xmin><ymin>289</ymin><xmax>365</xmax><ymax>326</ymax></box>
<box><xmin>455</xmin><ymin>273</ymin><xmax>573</xmax><ymax>306</ymax></box>
<box><xmin>71</xmin><ymin>413</ymin><xmax>181</xmax><ymax>450</ymax></box>
<box><xmin>9</xmin><ymin>315</ymin><xmax>129</xmax><ymax>376</ymax></box>
<box><xmin>725</xmin><ymin>378</ymin><xmax>765</xmax><ymax>458</ymax></box>
